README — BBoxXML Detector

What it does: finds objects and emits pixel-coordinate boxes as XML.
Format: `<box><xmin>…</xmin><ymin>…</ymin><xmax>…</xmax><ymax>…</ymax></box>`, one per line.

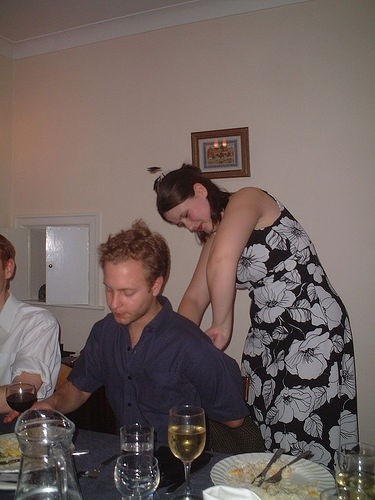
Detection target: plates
<box><xmin>0</xmin><ymin>434</ymin><xmax>75</xmax><ymax>473</ymax></box>
<box><xmin>210</xmin><ymin>452</ymin><xmax>335</xmax><ymax>500</ymax></box>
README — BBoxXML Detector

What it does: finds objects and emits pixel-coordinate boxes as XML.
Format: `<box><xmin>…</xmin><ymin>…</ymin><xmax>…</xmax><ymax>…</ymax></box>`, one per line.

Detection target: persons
<box><xmin>3</xmin><ymin>218</ymin><xmax>250</xmax><ymax>444</ymax></box>
<box><xmin>157</xmin><ymin>163</ymin><xmax>359</xmax><ymax>470</ymax></box>
<box><xmin>0</xmin><ymin>234</ymin><xmax>62</xmax><ymax>414</ymax></box>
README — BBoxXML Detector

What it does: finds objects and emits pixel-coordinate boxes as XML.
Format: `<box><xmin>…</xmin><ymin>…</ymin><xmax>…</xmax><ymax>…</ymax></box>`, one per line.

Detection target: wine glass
<box><xmin>6</xmin><ymin>382</ymin><xmax>37</xmax><ymax>430</ymax></box>
<box><xmin>168</xmin><ymin>405</ymin><xmax>206</xmax><ymax>500</ymax></box>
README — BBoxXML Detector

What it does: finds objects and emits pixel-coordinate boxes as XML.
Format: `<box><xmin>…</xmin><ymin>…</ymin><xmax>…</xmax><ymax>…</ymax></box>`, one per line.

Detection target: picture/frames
<box><xmin>191</xmin><ymin>127</ymin><xmax>250</xmax><ymax>178</ymax></box>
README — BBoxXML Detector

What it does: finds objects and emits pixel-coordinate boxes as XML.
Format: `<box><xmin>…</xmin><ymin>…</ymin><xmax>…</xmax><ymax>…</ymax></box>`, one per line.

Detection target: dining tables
<box><xmin>0</xmin><ymin>415</ymin><xmax>337</xmax><ymax>499</ymax></box>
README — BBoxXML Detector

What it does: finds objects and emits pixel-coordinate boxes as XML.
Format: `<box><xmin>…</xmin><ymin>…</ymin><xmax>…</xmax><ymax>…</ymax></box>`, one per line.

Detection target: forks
<box><xmin>261</xmin><ymin>450</ymin><xmax>312</xmax><ymax>483</ymax></box>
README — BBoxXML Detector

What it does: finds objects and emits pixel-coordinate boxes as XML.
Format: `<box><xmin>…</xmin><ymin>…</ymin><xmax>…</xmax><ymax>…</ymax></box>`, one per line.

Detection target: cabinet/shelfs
<box><xmin>0</xmin><ymin>212</ymin><xmax>104</xmax><ymax>311</ymax></box>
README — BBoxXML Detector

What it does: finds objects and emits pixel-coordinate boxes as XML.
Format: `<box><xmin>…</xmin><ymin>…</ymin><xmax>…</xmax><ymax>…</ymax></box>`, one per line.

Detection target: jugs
<box><xmin>15</xmin><ymin>408</ymin><xmax>83</xmax><ymax>499</ymax></box>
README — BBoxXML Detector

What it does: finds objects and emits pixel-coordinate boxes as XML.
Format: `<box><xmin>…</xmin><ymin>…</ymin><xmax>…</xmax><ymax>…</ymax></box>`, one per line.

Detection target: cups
<box><xmin>120</xmin><ymin>424</ymin><xmax>154</xmax><ymax>456</ymax></box>
<box><xmin>336</xmin><ymin>442</ymin><xmax>374</xmax><ymax>500</ymax></box>
<box><xmin>113</xmin><ymin>453</ymin><xmax>160</xmax><ymax>500</ymax></box>
